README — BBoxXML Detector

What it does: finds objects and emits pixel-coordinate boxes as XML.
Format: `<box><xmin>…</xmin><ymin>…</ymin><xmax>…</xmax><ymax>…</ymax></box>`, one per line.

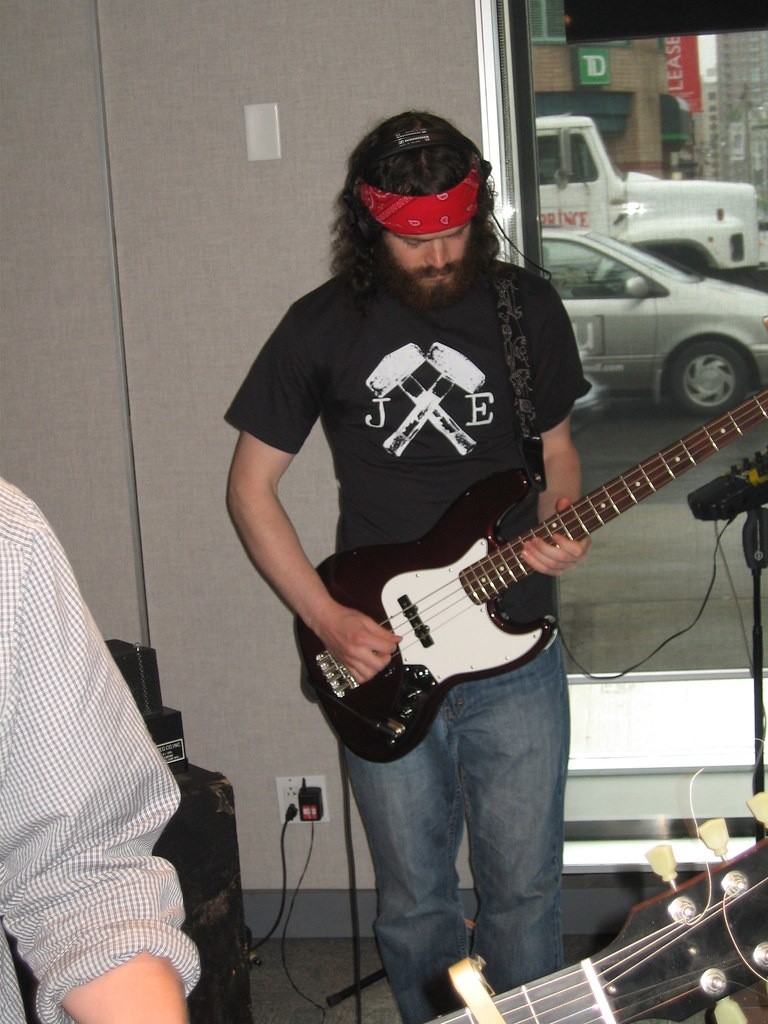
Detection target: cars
<box><xmin>540</xmin><ymin>225</ymin><xmax>768</xmax><ymax>419</ymax></box>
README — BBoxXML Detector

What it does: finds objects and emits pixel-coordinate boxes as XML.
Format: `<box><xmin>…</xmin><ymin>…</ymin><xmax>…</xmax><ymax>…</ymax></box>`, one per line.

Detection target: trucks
<box><xmin>534</xmin><ymin>111</ymin><xmax>756</xmax><ymax>288</ymax></box>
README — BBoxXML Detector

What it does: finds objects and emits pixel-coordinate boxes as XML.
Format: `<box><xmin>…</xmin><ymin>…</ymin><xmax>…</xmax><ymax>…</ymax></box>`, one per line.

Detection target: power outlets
<box><xmin>276</xmin><ymin>774</ymin><xmax>325</xmax><ymax>824</ymax></box>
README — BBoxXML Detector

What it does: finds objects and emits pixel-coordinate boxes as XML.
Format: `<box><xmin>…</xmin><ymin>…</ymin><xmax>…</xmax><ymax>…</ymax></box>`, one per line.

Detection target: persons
<box><xmin>0</xmin><ymin>473</ymin><xmax>202</xmax><ymax>1024</ymax></box>
<box><xmin>224</xmin><ymin>109</ymin><xmax>595</xmax><ymax>1024</ymax></box>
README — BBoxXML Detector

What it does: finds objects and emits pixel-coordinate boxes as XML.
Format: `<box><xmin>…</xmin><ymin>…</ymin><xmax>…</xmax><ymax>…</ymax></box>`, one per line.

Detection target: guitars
<box><xmin>420</xmin><ymin>787</ymin><xmax>768</xmax><ymax>1024</ymax></box>
<box><xmin>296</xmin><ymin>386</ymin><xmax>768</xmax><ymax>764</ymax></box>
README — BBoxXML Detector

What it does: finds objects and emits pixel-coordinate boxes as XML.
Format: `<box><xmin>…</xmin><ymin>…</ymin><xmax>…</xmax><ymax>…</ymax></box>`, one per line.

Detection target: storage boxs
<box><xmin>143</xmin><ymin>707</ymin><xmax>188</xmax><ymax>775</ymax></box>
<box><xmin>103</xmin><ymin>638</ymin><xmax>163</xmax><ymax>717</ymax></box>
